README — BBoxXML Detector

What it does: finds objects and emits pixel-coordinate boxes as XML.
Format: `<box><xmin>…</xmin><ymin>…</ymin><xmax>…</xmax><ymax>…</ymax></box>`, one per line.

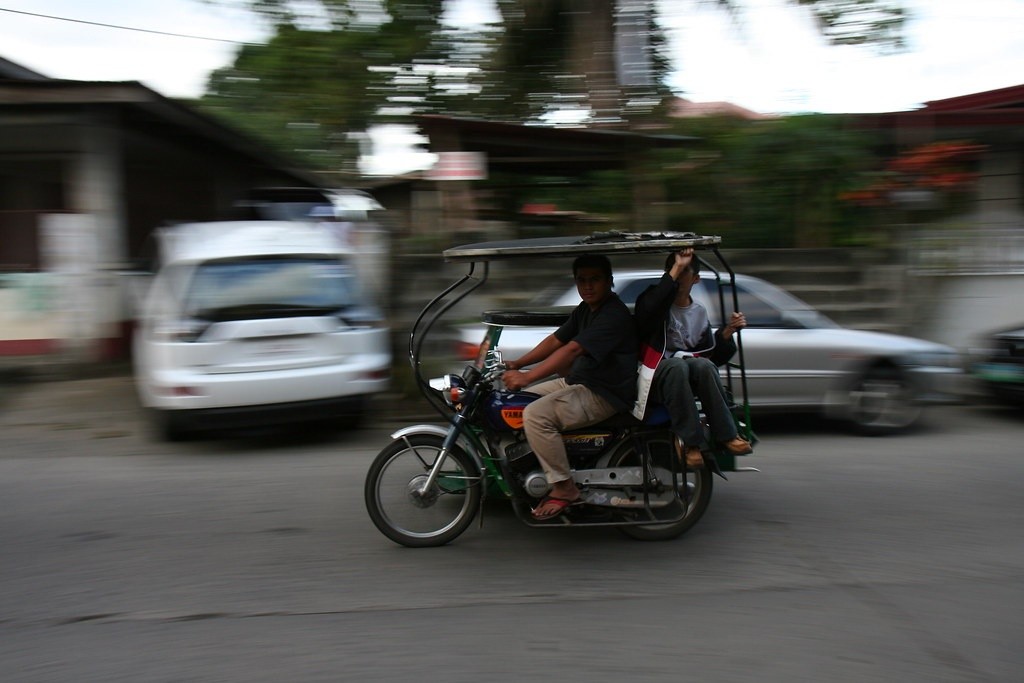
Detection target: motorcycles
<box><xmin>360</xmin><ymin>232</ymin><xmax>763</xmax><ymax>550</ymax></box>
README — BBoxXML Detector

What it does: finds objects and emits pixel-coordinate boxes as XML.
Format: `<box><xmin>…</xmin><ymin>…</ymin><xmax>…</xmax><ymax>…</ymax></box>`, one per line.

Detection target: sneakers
<box><xmin>674</xmin><ymin>435</ymin><xmax>704</xmax><ymax>469</ymax></box>
<box><xmin>724</xmin><ymin>435</ymin><xmax>753</xmax><ymax>456</ymax></box>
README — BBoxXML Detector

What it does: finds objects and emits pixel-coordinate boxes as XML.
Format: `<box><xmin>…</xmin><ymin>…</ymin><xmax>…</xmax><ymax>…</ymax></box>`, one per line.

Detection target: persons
<box><xmin>501</xmin><ymin>253</ymin><xmax>641</xmax><ymax>520</ymax></box>
<box><xmin>634</xmin><ymin>246</ymin><xmax>753</xmax><ymax>468</ymax></box>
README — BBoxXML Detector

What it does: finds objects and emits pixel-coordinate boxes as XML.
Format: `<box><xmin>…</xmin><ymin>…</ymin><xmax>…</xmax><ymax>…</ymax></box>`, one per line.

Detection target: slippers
<box><xmin>530</xmin><ymin>494</ymin><xmax>580</xmax><ymax>520</ymax></box>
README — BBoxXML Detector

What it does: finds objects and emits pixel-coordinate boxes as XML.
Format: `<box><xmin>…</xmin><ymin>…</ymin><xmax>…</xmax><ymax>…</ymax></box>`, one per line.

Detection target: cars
<box><xmin>443</xmin><ymin>270</ymin><xmax>961</xmax><ymax>434</ymax></box>
<box><xmin>135</xmin><ymin>213</ymin><xmax>396</xmax><ymax>441</ymax></box>
